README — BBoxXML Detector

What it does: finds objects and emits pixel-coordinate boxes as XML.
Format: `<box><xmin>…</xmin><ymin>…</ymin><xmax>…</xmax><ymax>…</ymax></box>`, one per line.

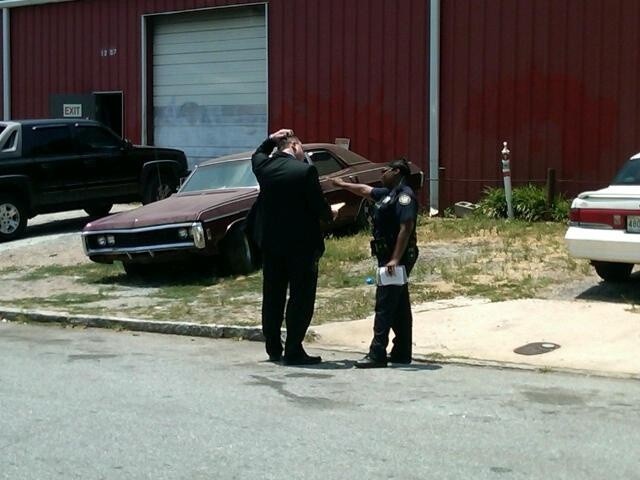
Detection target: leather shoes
<box><xmin>283</xmin><ymin>342</ymin><xmax>321</xmax><ymax>365</ymax></box>
<box><xmin>266</xmin><ymin>341</ymin><xmax>283</xmax><ymax>361</ymax></box>
<box><xmin>354</xmin><ymin>353</ymin><xmax>388</xmax><ymax>368</ymax></box>
<box><xmin>386</xmin><ymin>354</ymin><xmax>411</xmax><ymax>364</ymax></box>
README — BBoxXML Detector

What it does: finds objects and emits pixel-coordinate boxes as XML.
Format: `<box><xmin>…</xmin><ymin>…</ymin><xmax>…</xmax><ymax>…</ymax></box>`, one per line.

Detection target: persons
<box><xmin>243</xmin><ymin>128</ymin><xmax>333</xmax><ymax>365</ymax></box>
<box><xmin>326</xmin><ymin>158</ymin><xmax>420</xmax><ymax>368</ymax></box>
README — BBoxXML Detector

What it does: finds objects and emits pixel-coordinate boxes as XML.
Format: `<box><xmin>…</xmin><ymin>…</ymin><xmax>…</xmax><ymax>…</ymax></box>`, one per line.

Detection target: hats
<box><xmin>382</xmin><ymin>161</ymin><xmax>411</xmax><ymax>176</ymax></box>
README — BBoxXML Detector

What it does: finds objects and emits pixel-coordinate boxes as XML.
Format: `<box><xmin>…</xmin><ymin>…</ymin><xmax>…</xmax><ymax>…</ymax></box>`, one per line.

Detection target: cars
<box><xmin>77</xmin><ymin>137</ymin><xmax>427</xmax><ymax>282</ymax></box>
<box><xmin>561</xmin><ymin>148</ymin><xmax>640</xmax><ymax>284</ymax></box>
<box><xmin>0</xmin><ymin>116</ymin><xmax>194</xmax><ymax>245</ymax></box>
